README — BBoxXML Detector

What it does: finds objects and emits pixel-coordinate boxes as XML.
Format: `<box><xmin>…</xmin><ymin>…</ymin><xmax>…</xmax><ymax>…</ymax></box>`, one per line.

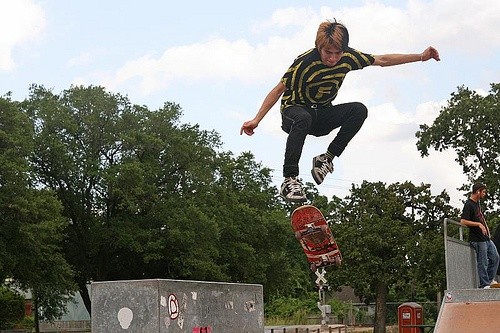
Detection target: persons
<box><xmin>240</xmin><ymin>17</ymin><xmax>439</xmax><ymax>202</ymax></box>
<box><xmin>460</xmin><ymin>182</ymin><xmax>500</xmax><ymax>288</ymax></box>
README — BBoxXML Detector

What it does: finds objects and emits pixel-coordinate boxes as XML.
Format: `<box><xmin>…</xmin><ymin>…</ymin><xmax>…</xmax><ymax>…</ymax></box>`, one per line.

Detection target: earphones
<box><xmin>478</xmin><ymin>192</ymin><xmax>480</xmax><ymax>194</ymax></box>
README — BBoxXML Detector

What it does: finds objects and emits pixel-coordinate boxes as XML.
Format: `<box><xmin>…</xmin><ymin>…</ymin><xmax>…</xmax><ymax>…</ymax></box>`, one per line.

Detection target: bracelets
<box><xmin>421</xmin><ymin>53</ymin><xmax>423</xmax><ymax>62</ymax></box>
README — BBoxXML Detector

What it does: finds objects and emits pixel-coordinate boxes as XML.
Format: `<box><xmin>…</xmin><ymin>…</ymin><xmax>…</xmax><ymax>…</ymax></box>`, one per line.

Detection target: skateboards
<box><xmin>290</xmin><ymin>204</ymin><xmax>343</xmax><ymax>272</ymax></box>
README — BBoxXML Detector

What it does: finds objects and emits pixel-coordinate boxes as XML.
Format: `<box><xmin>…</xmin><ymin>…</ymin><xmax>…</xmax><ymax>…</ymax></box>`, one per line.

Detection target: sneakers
<box><xmin>280</xmin><ymin>177</ymin><xmax>307</xmax><ymax>202</ymax></box>
<box><xmin>311</xmin><ymin>153</ymin><xmax>334</xmax><ymax>185</ymax></box>
